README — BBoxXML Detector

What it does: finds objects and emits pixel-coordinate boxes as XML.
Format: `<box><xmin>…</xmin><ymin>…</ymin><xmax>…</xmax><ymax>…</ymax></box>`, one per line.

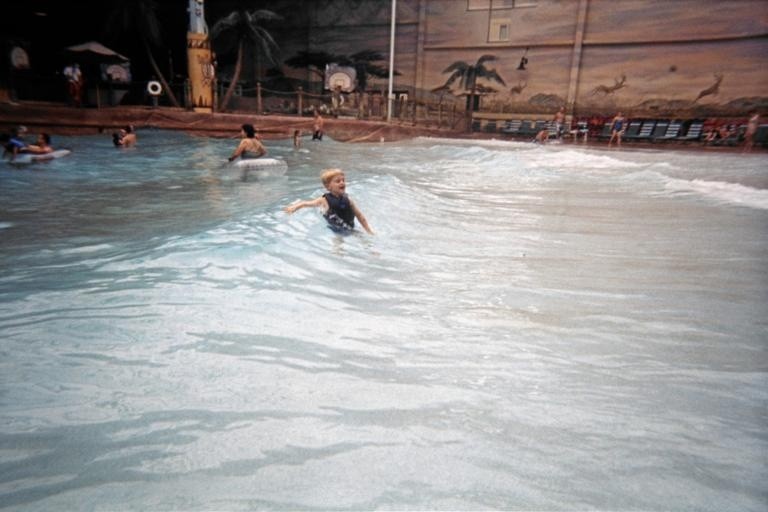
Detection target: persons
<box><xmin>227</xmin><ymin>123</ymin><xmax>267</xmax><ymax>162</ymax></box>
<box><xmin>281</xmin><ymin>167</ymin><xmax>374</xmax><ymax>234</ymax></box>
<box><xmin>112</xmin><ymin>124</ymin><xmax>136</xmax><ymax>149</ymax></box>
<box><xmin>98</xmin><ymin>126</ymin><xmax>107</xmax><ymax>135</ymax></box>
<box><xmin>2</xmin><ymin>124</ymin><xmax>53</xmax><ymax>163</ymax></box>
<box><xmin>310</xmin><ymin>109</ymin><xmax>325</xmax><ymax>141</ymax></box>
<box><xmin>608</xmin><ymin>111</ymin><xmax>626</xmax><ymax>149</ymax></box>
<box><xmin>703</xmin><ymin>109</ymin><xmax>760</xmax><ymax>152</ymax></box>
<box><xmin>294</xmin><ymin>129</ymin><xmax>300</xmax><ymax>148</ymax></box>
<box><xmin>532</xmin><ymin>128</ymin><xmax>549</xmax><ymax>144</ymax></box>
<box><xmin>551</xmin><ymin>106</ymin><xmax>566</xmax><ymax>141</ymax></box>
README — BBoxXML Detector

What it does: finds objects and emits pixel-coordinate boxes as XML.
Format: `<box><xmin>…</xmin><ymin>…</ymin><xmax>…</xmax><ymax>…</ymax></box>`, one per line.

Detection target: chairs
<box><xmin>471</xmin><ymin>119</ymin><xmax>768</xmax><ymax>147</ymax></box>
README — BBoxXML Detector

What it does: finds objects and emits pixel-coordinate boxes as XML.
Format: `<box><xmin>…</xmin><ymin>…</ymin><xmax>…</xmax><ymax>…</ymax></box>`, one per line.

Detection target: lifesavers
<box><xmin>146</xmin><ymin>80</ymin><xmax>161</xmax><ymax>94</ymax></box>
<box><xmin>3</xmin><ymin>148</ymin><xmax>70</xmax><ymax>166</ymax></box>
<box><xmin>280</xmin><ymin>99</ymin><xmax>294</xmax><ymax>112</ymax></box>
<box><xmin>211</xmin><ymin>154</ymin><xmax>289</xmax><ymax>185</ymax></box>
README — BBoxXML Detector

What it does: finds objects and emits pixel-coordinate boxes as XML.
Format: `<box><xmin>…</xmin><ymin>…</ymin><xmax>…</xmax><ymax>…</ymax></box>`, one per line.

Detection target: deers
<box><xmin>689</xmin><ymin>70</ymin><xmax>723</xmax><ymax>106</ymax></box>
<box><xmin>509</xmin><ymin>80</ymin><xmax>527</xmax><ymax>97</ymax></box>
<box><xmin>588</xmin><ymin>75</ymin><xmax>628</xmax><ymax>97</ymax></box>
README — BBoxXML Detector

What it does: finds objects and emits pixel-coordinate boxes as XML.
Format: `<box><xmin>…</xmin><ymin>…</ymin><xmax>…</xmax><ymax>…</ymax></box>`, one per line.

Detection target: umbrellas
<box><xmin>64</xmin><ymin>40</ymin><xmax>128</xmax><ymax>111</ymax></box>
